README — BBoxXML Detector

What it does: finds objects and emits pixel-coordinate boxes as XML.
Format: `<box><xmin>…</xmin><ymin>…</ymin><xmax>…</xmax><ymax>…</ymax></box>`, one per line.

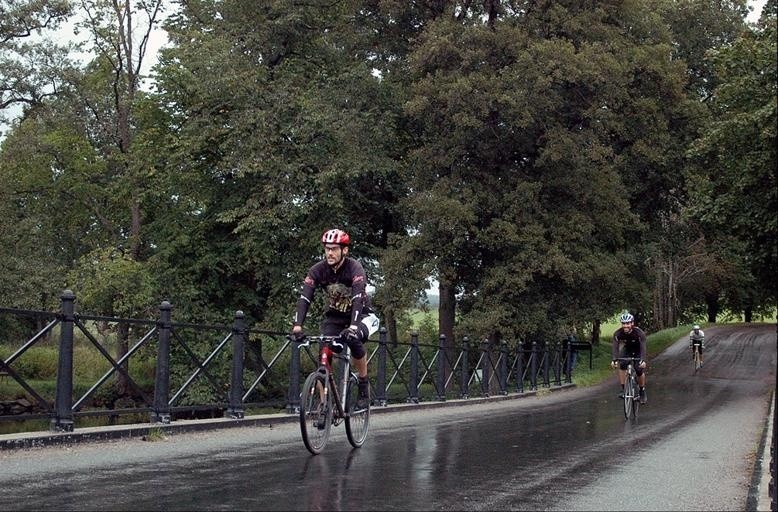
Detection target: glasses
<box><xmin>323</xmin><ymin>246</ymin><xmax>343</xmax><ymax>252</ymax></box>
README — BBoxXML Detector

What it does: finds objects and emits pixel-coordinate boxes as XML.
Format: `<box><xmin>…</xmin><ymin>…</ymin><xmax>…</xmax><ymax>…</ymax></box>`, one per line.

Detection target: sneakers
<box><xmin>618</xmin><ymin>389</ymin><xmax>624</xmax><ymax>398</ymax></box>
<box><xmin>357</xmin><ymin>376</ymin><xmax>370</xmax><ymax>409</ymax></box>
<box><xmin>639</xmin><ymin>386</ymin><xmax>647</xmax><ymax>404</ymax></box>
<box><xmin>317</xmin><ymin>402</ymin><xmax>330</xmax><ymax>429</ymax></box>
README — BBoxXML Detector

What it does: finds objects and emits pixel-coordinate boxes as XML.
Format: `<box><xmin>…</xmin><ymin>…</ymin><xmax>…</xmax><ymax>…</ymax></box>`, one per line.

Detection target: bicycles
<box><xmin>688</xmin><ymin>343</ymin><xmax>707</xmax><ymax>373</ymax></box>
<box><xmin>284</xmin><ymin>329</ymin><xmax>373</xmax><ymax>452</ymax></box>
<box><xmin>611</xmin><ymin>356</ymin><xmax>644</xmax><ymax>417</ymax></box>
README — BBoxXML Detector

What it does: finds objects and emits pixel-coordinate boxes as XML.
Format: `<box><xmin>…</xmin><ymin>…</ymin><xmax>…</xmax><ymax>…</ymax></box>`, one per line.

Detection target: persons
<box><xmin>690</xmin><ymin>325</ymin><xmax>705</xmax><ymax>368</ymax></box>
<box><xmin>611</xmin><ymin>312</ymin><xmax>648</xmax><ymax>405</ymax></box>
<box><xmin>292</xmin><ymin>228</ymin><xmax>380</xmax><ymax>430</ymax></box>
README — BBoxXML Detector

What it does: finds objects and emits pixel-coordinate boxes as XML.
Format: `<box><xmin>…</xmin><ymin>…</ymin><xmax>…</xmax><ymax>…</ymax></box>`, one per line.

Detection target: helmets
<box><xmin>323</xmin><ymin>229</ymin><xmax>350</xmax><ymax>245</ymax></box>
<box><xmin>693</xmin><ymin>325</ymin><xmax>700</xmax><ymax>330</ymax></box>
<box><xmin>620</xmin><ymin>313</ymin><xmax>634</xmax><ymax>323</ymax></box>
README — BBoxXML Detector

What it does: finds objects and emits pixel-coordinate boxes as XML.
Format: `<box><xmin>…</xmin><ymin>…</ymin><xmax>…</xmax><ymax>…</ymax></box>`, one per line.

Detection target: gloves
<box><xmin>339</xmin><ymin>328</ymin><xmax>358</xmax><ymax>343</ymax></box>
<box><xmin>292</xmin><ymin>330</ymin><xmax>306</xmax><ymax>342</ymax></box>
